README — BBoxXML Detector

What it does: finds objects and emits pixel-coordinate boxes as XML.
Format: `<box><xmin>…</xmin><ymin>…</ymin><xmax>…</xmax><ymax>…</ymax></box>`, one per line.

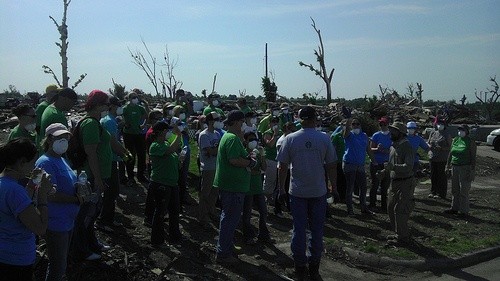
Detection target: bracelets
<box><xmin>39</xmin><ymin>203</ymin><xmax>49</xmax><ymax>206</ymax></box>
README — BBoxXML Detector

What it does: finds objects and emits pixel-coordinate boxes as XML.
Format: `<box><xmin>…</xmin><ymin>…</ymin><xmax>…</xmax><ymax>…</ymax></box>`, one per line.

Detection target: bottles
<box><xmin>177</xmin><ymin>146</ymin><xmax>187</xmax><ymax>161</ymax></box>
<box><xmin>78</xmin><ymin>171</ymin><xmax>87</xmax><ymax>198</ymax></box>
<box><xmin>165</xmin><ymin>130</ymin><xmax>172</xmax><ymax>141</ymax></box>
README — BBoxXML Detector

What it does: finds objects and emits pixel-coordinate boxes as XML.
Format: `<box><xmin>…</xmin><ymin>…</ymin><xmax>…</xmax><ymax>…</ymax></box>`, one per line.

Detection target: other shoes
<box><xmin>84</xmin><ymin>166</ymin><xmax>471</xmax><ymax>281</ymax></box>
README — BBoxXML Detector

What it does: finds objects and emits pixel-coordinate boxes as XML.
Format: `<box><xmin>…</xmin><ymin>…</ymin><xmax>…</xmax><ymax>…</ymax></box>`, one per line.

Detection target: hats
<box><xmin>46</xmin><ymin>84</ymin><xmax>63</xmax><ymax>94</ymax></box>
<box><xmin>87</xmin><ymin>90</ymin><xmax>109</xmax><ymax>106</ymax></box>
<box><xmin>46</xmin><ymin>122</ymin><xmax>73</xmax><ymax>138</ymax></box>
<box><xmin>128</xmin><ymin>91</ymin><xmax>140</xmax><ymax>100</ymax></box>
<box><xmin>108</xmin><ymin>96</ymin><xmax>125</xmax><ymax>105</ymax></box>
<box><xmin>153</xmin><ymin>120</ymin><xmax>173</xmax><ymax>130</ymax></box>
<box><xmin>203</xmin><ymin>94</ymin><xmax>446</xmax><ymax>128</ymax></box>
<box><xmin>163</xmin><ymin>102</ymin><xmax>175</xmax><ymax>108</ymax></box>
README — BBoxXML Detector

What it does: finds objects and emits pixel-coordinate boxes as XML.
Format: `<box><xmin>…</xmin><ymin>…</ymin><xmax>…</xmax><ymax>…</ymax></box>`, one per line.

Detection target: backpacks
<box><xmin>67</xmin><ymin>115</ymin><xmax>103</xmax><ymax>166</ymax></box>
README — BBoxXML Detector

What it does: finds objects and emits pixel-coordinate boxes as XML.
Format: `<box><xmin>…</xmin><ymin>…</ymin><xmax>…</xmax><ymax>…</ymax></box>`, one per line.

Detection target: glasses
<box><xmin>21</xmin><ymin>114</ymin><xmax>37</xmax><ymax>118</ymax></box>
<box><xmin>247</xmin><ymin>138</ymin><xmax>259</xmax><ymax>142</ymax></box>
<box><xmin>102</xmin><ymin>103</ymin><xmax>110</xmax><ymax>107</ymax></box>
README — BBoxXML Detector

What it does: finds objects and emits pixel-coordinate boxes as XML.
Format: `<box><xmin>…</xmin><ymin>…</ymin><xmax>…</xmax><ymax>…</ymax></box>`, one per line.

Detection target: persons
<box><xmin>427</xmin><ymin>120</ymin><xmax>452</xmax><ymax>199</ymax></box>
<box><xmin>6</xmin><ymin>86</ymin><xmax>77</xmax><ymax>187</ymax></box>
<box><xmin>369</xmin><ymin>116</ymin><xmax>395</xmax><ymax>213</ymax></box>
<box><xmin>329</xmin><ymin>117</ymin><xmax>373</xmax><ymax>218</ymax></box>
<box><xmin>0</xmin><ymin>138</ymin><xmax>53</xmax><ymax>281</ymax></box>
<box><xmin>375</xmin><ymin>122</ymin><xmax>418</xmax><ymax>248</ymax></box>
<box><xmin>444</xmin><ymin>124</ymin><xmax>476</xmax><ymax>218</ymax></box>
<box><xmin>274</xmin><ymin>106</ymin><xmax>338</xmax><ymax>281</ymax></box>
<box><xmin>405</xmin><ymin>121</ymin><xmax>434</xmax><ymax>165</ymax></box>
<box><xmin>74</xmin><ymin>90</ymin><xmax>112</xmax><ymax>262</ymax></box>
<box><xmin>32</xmin><ymin>122</ymin><xmax>91</xmax><ymax>281</ymax></box>
<box><xmin>97</xmin><ymin>90</ymin><xmax>196</xmax><ymax>253</ymax></box>
<box><xmin>211</xmin><ymin>109</ymin><xmax>258</xmax><ymax>266</ymax></box>
<box><xmin>198</xmin><ymin>92</ymin><xmax>303</xmax><ymax>246</ymax></box>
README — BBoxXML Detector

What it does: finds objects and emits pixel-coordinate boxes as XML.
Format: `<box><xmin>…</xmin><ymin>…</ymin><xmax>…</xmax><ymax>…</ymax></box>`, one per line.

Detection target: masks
<box><xmin>168</xmin><ymin>110</ymin><xmax>172</xmax><ymax>116</ymax></box>
<box><xmin>166</xmin><ymin>132</ymin><xmax>172</xmax><ymax>139</ymax></box>
<box><xmin>100</xmin><ymin>111</ymin><xmax>109</xmax><ymax>118</ymax></box>
<box><xmin>179</xmin><ymin>113</ymin><xmax>186</xmax><ymax>121</ymax></box>
<box><xmin>52</xmin><ymin>138</ymin><xmax>68</xmax><ymax>154</ymax></box>
<box><xmin>24</xmin><ymin>122</ymin><xmax>36</xmax><ymax>131</ymax></box>
<box><xmin>213</xmin><ymin>119</ymin><xmax>466</xmax><ymax>150</ymax></box>
<box><xmin>115</xmin><ymin>107</ymin><xmax>124</xmax><ymax>116</ymax></box>
